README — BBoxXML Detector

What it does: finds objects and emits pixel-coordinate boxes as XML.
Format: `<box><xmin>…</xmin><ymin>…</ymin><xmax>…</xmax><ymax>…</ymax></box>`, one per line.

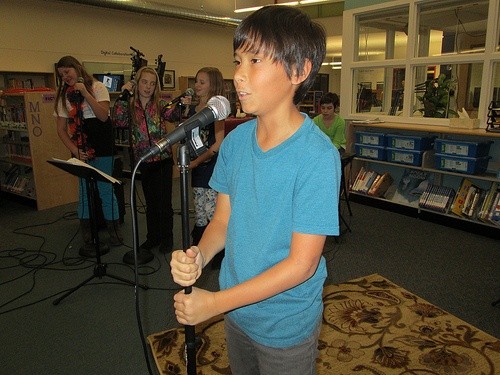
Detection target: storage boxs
<box><xmin>354</xmin><ymin>131</ymin><xmax>493</xmax><ymax>175</ymax></box>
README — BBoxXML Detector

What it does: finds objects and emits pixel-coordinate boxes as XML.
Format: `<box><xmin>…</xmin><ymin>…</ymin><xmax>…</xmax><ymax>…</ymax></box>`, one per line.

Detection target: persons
<box><xmin>176</xmin><ymin>67</ymin><xmax>225</xmax><ymax>270</ymax></box>
<box><xmin>114</xmin><ymin>67</ymin><xmax>191</xmax><ymax>254</ymax></box>
<box><xmin>169</xmin><ymin>5</ymin><xmax>349</xmax><ymax>375</ymax></box>
<box><xmin>51</xmin><ymin>55</ymin><xmax>124</xmax><ymax>246</ymax></box>
<box><xmin>310</xmin><ymin>93</ymin><xmax>346</xmax><ymax>161</ymax></box>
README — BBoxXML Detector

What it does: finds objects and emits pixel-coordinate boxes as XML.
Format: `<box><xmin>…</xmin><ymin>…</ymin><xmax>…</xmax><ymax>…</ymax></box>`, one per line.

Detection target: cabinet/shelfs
<box><xmin>345</xmin><ymin>121</ymin><xmax>500</xmax><ymax>230</ymax></box>
<box><xmin>179</xmin><ymin>76</ymin><xmax>256</xmax><ymax>138</ymax></box>
<box><xmin>0</xmin><ymin>91</ymin><xmax>80</xmax><ymax>211</ymax></box>
<box><xmin>107</xmin><ymin>90</ymin><xmax>182</xmax><ymax>178</ymax></box>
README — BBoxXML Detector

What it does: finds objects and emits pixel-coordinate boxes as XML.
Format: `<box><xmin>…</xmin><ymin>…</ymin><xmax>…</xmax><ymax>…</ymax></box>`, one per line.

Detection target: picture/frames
<box><xmin>163</xmin><ymin>70</ymin><xmax>175</xmax><ymax>88</ymax></box>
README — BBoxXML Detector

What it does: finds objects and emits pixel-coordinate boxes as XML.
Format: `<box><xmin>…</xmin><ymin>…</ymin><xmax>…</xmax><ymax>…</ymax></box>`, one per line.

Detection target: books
<box><xmin>349</xmin><ymin>130</ymin><xmax>500</xmax><ymax>225</ymax></box>
<box><xmin>0</xmin><ymin>76</ymin><xmax>32</xmax><ymax>193</ymax></box>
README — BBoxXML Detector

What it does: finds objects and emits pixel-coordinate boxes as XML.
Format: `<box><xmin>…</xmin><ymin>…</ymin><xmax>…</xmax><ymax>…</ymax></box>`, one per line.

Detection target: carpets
<box><xmin>145</xmin><ymin>273</ymin><xmax>500</xmax><ymax>375</ymax></box>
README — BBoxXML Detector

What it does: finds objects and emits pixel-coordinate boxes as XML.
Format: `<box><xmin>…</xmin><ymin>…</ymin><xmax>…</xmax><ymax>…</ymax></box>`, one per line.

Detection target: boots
<box><xmin>211</xmin><ymin>250</ymin><xmax>224</xmax><ymax>269</ymax></box>
<box><xmin>108</xmin><ymin>220</ymin><xmax>123</xmax><ymax>247</ymax></box>
<box><xmin>192</xmin><ymin>220</ymin><xmax>210</xmax><ymax>245</ymax></box>
<box><xmin>80</xmin><ymin>219</ymin><xmax>93</xmax><ymax>243</ymax></box>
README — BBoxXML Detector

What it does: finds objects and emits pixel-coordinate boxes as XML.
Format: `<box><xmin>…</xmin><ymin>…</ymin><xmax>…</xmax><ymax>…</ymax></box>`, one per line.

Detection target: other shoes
<box><xmin>160</xmin><ymin>232</ymin><xmax>173</xmax><ymax>254</ymax></box>
<box><xmin>139</xmin><ymin>240</ymin><xmax>158</xmax><ymax>251</ymax></box>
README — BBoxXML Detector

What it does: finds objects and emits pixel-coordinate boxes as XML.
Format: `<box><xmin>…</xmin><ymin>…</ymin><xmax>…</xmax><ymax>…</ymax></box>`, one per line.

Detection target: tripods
<box><xmin>46</xmin><ymin>157</ymin><xmax>150</xmax><ymax>305</ymax></box>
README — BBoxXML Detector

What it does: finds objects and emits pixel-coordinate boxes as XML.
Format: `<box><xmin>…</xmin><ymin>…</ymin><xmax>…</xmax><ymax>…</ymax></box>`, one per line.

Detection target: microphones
<box><xmin>167</xmin><ymin>88</ymin><xmax>194</xmax><ymax>106</ymax></box>
<box><xmin>140</xmin><ymin>96</ymin><xmax>231</xmax><ymax>162</ymax></box>
<box><xmin>120</xmin><ymin>80</ymin><xmax>136</xmax><ymax>99</ymax></box>
<box><xmin>76</xmin><ymin>76</ymin><xmax>84</xmax><ymax>96</ymax></box>
